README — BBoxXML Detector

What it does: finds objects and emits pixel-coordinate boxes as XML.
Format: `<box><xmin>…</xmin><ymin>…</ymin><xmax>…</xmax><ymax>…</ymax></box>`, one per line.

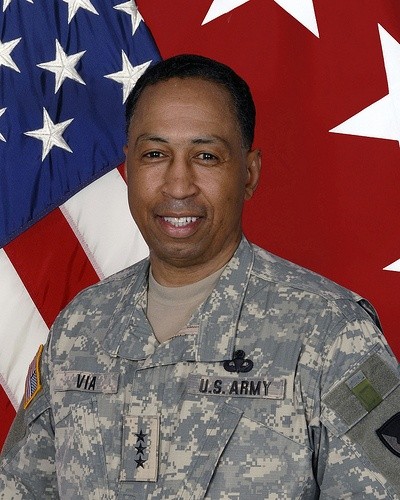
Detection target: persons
<box><xmin>0</xmin><ymin>52</ymin><xmax>399</xmax><ymax>499</ymax></box>
